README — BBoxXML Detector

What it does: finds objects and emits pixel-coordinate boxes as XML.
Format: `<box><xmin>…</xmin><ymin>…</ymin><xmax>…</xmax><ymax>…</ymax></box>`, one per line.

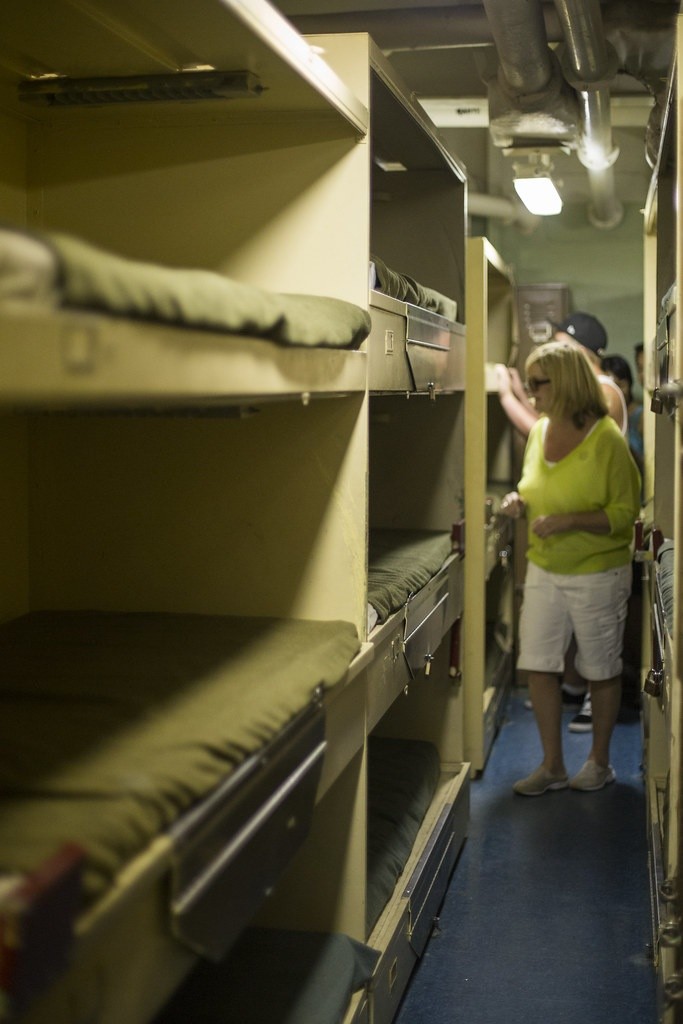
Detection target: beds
<box><xmin>0</xmin><ymin>0</ymin><xmax>683</xmax><ymax>1024</ymax></box>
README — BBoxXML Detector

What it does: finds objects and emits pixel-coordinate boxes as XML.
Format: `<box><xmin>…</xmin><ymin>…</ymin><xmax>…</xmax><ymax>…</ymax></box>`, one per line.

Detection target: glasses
<box><xmin>526</xmin><ymin>373</ymin><xmax>550</xmax><ymax>392</ymax></box>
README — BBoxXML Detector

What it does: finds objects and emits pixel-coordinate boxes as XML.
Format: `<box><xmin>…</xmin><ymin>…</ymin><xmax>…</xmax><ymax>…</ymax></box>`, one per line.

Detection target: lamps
<box><xmin>513</xmin><ymin>165</ymin><xmax>564</xmax><ymax>217</ymax></box>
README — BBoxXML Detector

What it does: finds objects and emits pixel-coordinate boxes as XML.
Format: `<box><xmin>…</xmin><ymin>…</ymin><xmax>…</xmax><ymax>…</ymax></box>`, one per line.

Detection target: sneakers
<box><xmin>568</xmin><ymin>692</ymin><xmax>593</xmax><ymax>732</ymax></box>
<box><xmin>569</xmin><ymin>760</ymin><xmax>616</xmax><ymax>791</ymax></box>
<box><xmin>512</xmin><ymin>766</ymin><xmax>568</xmax><ymax>795</ymax></box>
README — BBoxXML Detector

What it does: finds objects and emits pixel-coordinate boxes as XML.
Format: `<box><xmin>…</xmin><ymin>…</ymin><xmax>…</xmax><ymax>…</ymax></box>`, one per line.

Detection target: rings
<box><xmin>503</xmin><ymin>501</ymin><xmax>510</xmax><ymax>508</ymax></box>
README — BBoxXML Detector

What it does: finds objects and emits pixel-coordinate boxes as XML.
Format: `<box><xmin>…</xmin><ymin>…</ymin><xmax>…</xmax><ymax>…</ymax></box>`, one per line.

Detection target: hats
<box><xmin>545</xmin><ymin>312</ymin><xmax>608</xmax><ymax>357</ymax></box>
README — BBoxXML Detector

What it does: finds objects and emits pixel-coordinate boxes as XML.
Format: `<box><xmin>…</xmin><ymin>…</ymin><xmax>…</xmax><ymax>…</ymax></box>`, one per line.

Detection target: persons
<box><xmin>501</xmin><ymin>342</ymin><xmax>643</xmax><ymax>796</ymax></box>
<box><xmin>496</xmin><ymin>311</ymin><xmax>644</xmax><ymax>732</ymax></box>
